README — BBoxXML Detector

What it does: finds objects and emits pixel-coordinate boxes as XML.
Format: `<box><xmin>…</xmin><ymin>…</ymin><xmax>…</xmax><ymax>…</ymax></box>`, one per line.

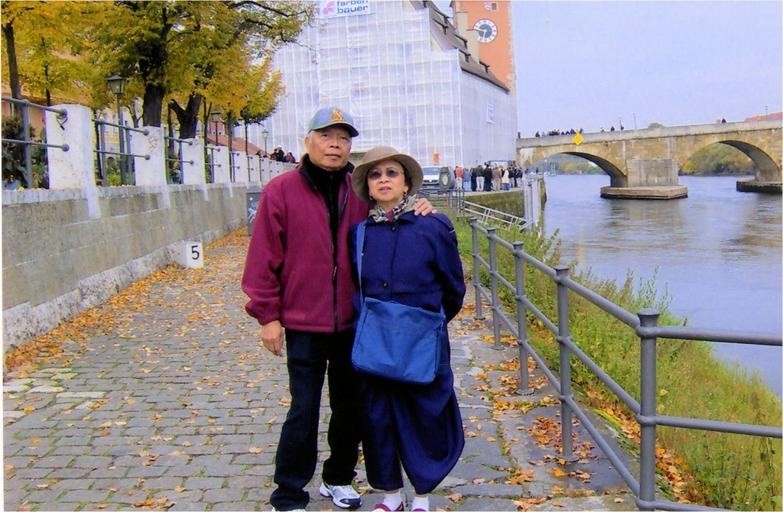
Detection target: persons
<box><xmin>451</xmin><ymin>159</ymin><xmax>541</xmax><ymax>193</ymax></box>
<box><xmin>241</xmin><ymin>106</ymin><xmax>439</xmax><ymax>511</ymax></box>
<box><xmin>516</xmin><ymin>125</ymin><xmax>624</xmax><ymax>138</ymax></box>
<box><xmin>344</xmin><ymin>144</ymin><xmax>468</xmax><ymax>510</ymax></box>
<box><xmin>255</xmin><ymin>146</ymin><xmax>296</xmax><ymax>165</ymax></box>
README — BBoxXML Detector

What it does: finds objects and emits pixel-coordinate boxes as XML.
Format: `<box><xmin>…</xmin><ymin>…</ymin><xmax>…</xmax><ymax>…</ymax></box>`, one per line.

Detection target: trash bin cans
<box><xmin>245</xmin><ymin>191</ymin><xmax>263</xmax><ymax>236</ymax></box>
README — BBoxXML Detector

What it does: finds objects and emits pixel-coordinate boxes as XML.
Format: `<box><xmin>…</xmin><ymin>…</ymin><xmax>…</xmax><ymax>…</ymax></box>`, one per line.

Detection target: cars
<box><xmin>422</xmin><ymin>165</ymin><xmax>457</xmax><ymax>192</ymax></box>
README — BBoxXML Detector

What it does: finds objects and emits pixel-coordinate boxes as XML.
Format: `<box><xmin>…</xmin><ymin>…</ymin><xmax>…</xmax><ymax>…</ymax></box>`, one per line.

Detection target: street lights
<box><xmin>105</xmin><ymin>72</ymin><xmax>130</xmax><ymax>186</ymax></box>
<box><xmin>261</xmin><ymin>127</ymin><xmax>270</xmax><ymax>158</ymax></box>
<box><xmin>210</xmin><ymin>107</ymin><xmax>222</xmax><ymax>146</ymax></box>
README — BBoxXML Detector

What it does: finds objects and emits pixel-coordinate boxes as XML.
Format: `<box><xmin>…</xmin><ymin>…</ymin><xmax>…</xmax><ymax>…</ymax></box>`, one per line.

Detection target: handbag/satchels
<box><xmin>350</xmin><ymin>296</ymin><xmax>445</xmax><ymax>385</ymax></box>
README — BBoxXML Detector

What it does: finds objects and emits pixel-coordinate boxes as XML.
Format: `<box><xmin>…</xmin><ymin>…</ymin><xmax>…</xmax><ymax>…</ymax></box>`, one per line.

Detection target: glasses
<box><xmin>367</xmin><ymin>167</ymin><xmax>402</xmax><ymax>181</ymax></box>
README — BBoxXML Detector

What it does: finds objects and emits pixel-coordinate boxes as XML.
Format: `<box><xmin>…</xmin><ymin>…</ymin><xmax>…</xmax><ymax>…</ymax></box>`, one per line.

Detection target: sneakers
<box><xmin>319</xmin><ymin>480</ymin><xmax>363</xmax><ymax>509</ymax></box>
<box><xmin>371</xmin><ymin>501</ymin><xmax>405</xmax><ymax>512</ymax></box>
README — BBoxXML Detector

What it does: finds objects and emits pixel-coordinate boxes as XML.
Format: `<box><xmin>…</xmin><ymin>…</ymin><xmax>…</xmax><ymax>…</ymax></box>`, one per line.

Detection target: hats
<box><xmin>351</xmin><ymin>146</ymin><xmax>424</xmax><ymax>202</ymax></box>
<box><xmin>306</xmin><ymin>107</ymin><xmax>359</xmax><ymax>137</ymax></box>
